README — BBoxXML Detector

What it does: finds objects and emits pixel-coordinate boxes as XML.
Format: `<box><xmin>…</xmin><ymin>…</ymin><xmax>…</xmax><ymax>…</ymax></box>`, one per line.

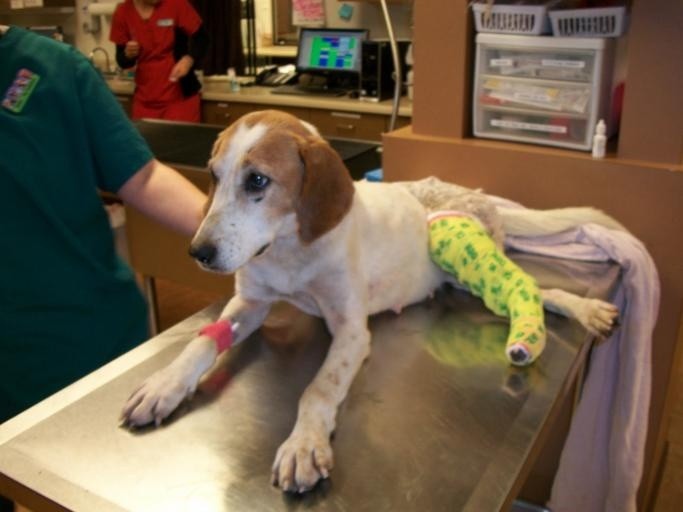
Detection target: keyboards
<box><xmin>271</xmin><ymin>84</ymin><xmax>346</xmax><ymax>97</ymax></box>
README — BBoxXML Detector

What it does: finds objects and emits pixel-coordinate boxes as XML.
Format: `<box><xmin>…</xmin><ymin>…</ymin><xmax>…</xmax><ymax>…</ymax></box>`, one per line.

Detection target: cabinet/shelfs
<box><xmin>105</xmin><ymin>82</ymin><xmax>412</xmax><ymax>147</ymax></box>
<box><xmin>472</xmin><ymin>33</ymin><xmax>617</xmax><ymax>152</ymax></box>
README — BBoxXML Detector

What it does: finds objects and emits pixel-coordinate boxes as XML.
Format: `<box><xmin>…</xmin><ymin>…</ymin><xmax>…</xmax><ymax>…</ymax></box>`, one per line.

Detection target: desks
<box><xmin>0</xmin><ymin>249</ymin><xmax>622</xmax><ymax>512</ymax></box>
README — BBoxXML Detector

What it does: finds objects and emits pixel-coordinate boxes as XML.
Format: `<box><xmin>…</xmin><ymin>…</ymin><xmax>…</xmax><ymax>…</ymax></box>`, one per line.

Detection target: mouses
<box><xmin>349</xmin><ymin>90</ymin><xmax>359</xmax><ymax>99</ymax></box>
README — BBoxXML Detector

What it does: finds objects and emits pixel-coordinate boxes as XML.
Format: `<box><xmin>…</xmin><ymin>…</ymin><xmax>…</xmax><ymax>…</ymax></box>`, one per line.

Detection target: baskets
<box><xmin>471</xmin><ymin>1</ymin><xmax>551</xmax><ymax>36</ymax></box>
<box><xmin>547</xmin><ymin>3</ymin><xmax>629</xmax><ymax>38</ymax></box>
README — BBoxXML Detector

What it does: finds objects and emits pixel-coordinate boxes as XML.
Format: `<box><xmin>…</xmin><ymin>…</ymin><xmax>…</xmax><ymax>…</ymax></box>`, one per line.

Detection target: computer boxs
<box><xmin>361</xmin><ymin>39</ymin><xmax>412</xmax><ymax>102</ymax></box>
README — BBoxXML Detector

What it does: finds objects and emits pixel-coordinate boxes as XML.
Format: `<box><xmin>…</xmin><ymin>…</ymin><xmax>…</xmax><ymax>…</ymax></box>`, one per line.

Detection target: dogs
<box><xmin>117</xmin><ymin>106</ymin><xmax>622</xmax><ymax>499</ymax></box>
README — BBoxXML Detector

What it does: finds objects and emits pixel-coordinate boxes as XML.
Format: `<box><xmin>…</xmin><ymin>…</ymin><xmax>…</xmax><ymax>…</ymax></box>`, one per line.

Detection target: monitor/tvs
<box><xmin>295</xmin><ymin>27</ymin><xmax>370</xmax><ymax>92</ymax></box>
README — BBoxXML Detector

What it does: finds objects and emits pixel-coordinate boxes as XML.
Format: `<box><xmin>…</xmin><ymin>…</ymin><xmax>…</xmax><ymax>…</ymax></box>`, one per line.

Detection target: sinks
<box><xmin>100</xmin><ymin>74</ymin><xmax>114</xmax><ymax>82</ymax></box>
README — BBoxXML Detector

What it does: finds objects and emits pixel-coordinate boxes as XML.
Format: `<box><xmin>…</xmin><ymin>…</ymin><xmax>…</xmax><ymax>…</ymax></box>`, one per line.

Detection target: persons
<box><xmin>110</xmin><ymin>1</ymin><xmax>204</xmax><ymax>123</ymax></box>
<box><xmin>0</xmin><ymin>22</ymin><xmax>209</xmax><ymax>424</ymax></box>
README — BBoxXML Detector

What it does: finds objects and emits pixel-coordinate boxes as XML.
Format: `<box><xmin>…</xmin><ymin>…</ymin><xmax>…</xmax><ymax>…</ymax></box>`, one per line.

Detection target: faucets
<box><xmin>88</xmin><ymin>46</ymin><xmax>110</xmax><ymax>71</ymax></box>
